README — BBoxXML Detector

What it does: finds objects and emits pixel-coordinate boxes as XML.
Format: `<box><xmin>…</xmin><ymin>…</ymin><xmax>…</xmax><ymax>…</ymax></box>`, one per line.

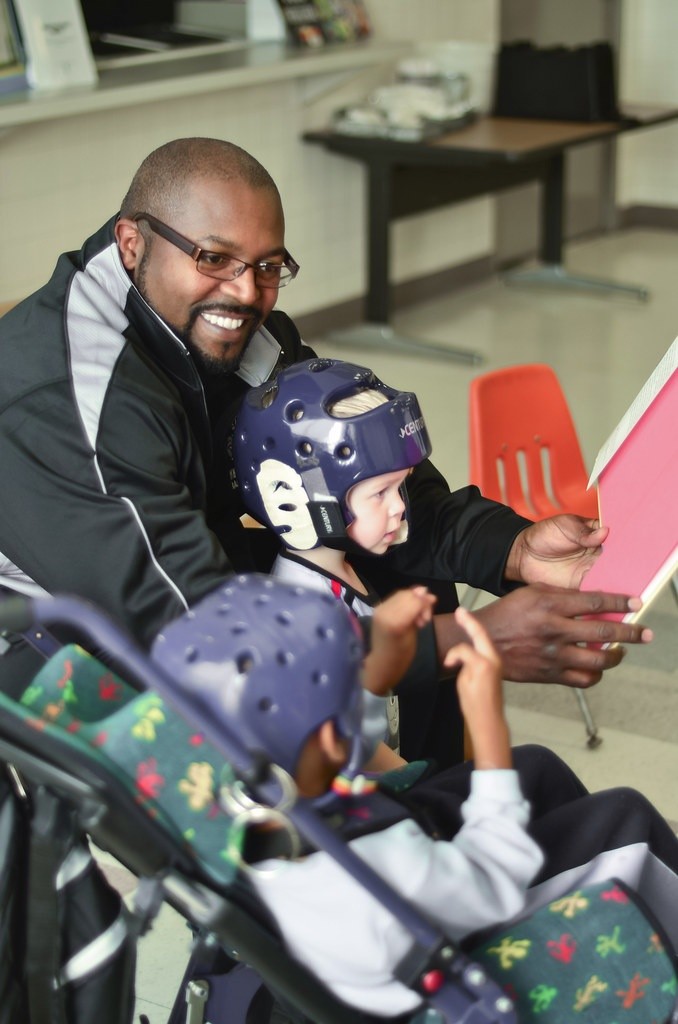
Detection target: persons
<box><xmin>151</xmin><ymin>573</ymin><xmax>677</xmax><ymax>1019</ymax></box>
<box><xmin>0</xmin><ymin>136</ymin><xmax>656</xmax><ymax>755</ymax></box>
<box><xmin>229</xmin><ymin>356</ymin><xmax>431</xmax><ymax>616</ymax></box>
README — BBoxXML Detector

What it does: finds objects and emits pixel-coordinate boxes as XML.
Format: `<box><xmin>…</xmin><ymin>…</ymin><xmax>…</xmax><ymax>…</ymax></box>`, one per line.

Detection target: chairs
<box><xmin>468</xmin><ymin>362</ymin><xmax>678</xmax><ymax>751</ymax></box>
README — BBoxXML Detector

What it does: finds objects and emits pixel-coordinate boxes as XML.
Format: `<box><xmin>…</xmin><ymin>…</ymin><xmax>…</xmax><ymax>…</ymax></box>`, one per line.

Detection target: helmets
<box><xmin>148</xmin><ymin>575</ymin><xmax>363</xmax><ymax>816</ymax></box>
<box><xmin>228</xmin><ymin>357</ymin><xmax>430</xmax><ymax>551</ymax></box>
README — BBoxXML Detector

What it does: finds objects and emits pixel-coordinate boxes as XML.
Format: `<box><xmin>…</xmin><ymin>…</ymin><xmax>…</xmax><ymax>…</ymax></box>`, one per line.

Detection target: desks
<box><xmin>302</xmin><ymin>100</ymin><xmax>678</xmax><ymax>368</ymax></box>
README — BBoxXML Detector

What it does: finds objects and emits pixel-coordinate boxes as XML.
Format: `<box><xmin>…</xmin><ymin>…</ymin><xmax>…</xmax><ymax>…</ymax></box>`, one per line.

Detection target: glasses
<box><xmin>131</xmin><ymin>212</ymin><xmax>299</xmax><ymax>289</ymax></box>
